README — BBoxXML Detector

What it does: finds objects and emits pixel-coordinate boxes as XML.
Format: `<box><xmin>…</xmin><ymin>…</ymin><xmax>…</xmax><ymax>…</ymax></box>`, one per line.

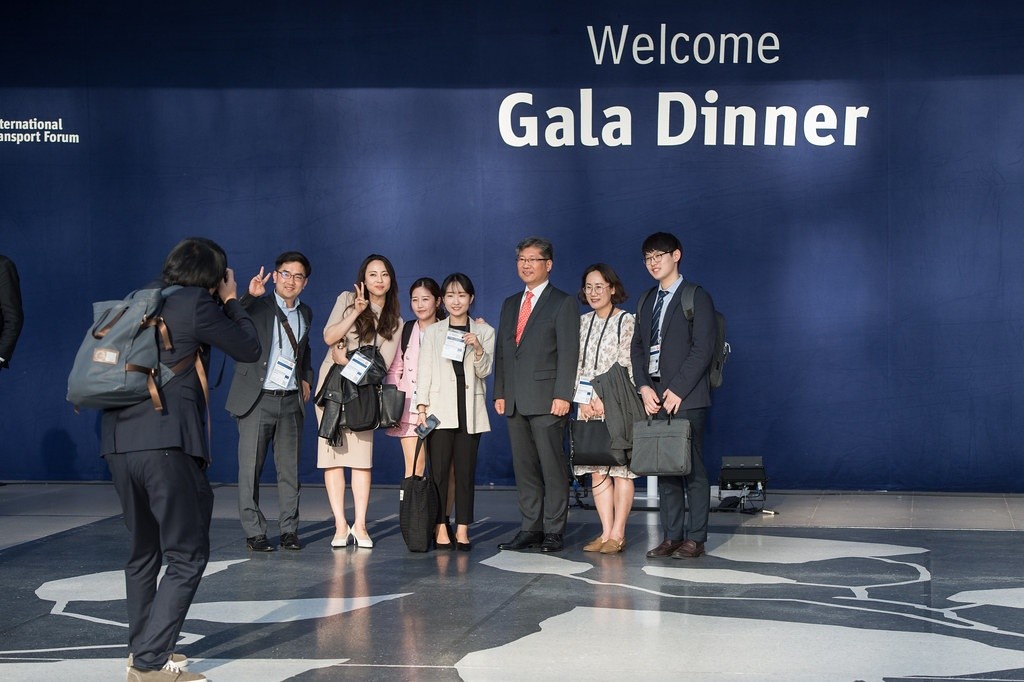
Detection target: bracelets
<box><xmin>418</xmin><ymin>412</ymin><xmax>427</xmax><ymax>415</ymax></box>
<box><xmin>475</xmin><ymin>351</ymin><xmax>484</xmax><ymax>356</ymax></box>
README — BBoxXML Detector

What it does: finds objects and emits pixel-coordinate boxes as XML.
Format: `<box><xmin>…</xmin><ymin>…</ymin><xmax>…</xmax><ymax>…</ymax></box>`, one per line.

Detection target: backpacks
<box><xmin>638</xmin><ymin>286</ymin><xmax>731</xmax><ymax>389</ymax></box>
<box><xmin>66</xmin><ymin>284</ymin><xmax>198</xmax><ymax>410</ymax></box>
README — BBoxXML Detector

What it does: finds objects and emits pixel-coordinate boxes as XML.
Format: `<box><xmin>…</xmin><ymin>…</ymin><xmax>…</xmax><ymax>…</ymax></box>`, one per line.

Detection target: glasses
<box><xmin>516</xmin><ymin>256</ymin><xmax>548</xmax><ymax>265</ymax></box>
<box><xmin>582</xmin><ymin>284</ymin><xmax>610</xmax><ymax>295</ymax></box>
<box><xmin>277</xmin><ymin>269</ymin><xmax>307</xmax><ymax>281</ymax></box>
<box><xmin>641</xmin><ymin>251</ymin><xmax>671</xmax><ymax>266</ymax></box>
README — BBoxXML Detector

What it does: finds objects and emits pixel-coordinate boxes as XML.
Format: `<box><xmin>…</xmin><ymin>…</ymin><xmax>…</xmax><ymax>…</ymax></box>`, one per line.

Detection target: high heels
<box><xmin>433</xmin><ymin>532</ymin><xmax>472</xmax><ymax>552</ymax></box>
<box><xmin>331</xmin><ymin>523</ymin><xmax>373</xmax><ymax>548</ymax></box>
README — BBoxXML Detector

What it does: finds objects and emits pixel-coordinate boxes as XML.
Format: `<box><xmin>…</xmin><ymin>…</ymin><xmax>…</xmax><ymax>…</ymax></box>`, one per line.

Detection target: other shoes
<box><xmin>583</xmin><ymin>537</ymin><xmax>628</xmax><ymax>554</ymax></box>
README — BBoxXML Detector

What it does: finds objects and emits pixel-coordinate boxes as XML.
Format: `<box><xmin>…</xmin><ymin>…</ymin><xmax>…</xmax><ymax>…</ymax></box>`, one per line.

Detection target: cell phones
<box><xmin>414</xmin><ymin>414</ymin><xmax>441</xmax><ymax>439</ymax></box>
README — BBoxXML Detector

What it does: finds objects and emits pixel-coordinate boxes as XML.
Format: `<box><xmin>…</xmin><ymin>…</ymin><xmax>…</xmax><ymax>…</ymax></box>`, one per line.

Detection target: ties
<box><xmin>649</xmin><ymin>290</ymin><xmax>669</xmax><ymax>347</ymax></box>
<box><xmin>516</xmin><ymin>292</ymin><xmax>534</xmax><ymax>344</ymax></box>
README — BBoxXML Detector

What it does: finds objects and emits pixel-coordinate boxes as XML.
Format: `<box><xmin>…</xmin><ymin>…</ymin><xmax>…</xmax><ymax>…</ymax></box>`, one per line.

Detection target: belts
<box><xmin>261</xmin><ymin>389</ymin><xmax>298</xmax><ymax>396</ymax></box>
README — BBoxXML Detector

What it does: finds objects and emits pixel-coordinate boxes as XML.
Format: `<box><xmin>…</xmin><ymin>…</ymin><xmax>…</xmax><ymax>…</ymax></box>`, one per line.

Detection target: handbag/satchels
<box><xmin>630</xmin><ymin>403</ymin><xmax>692</xmax><ymax>477</ymax></box>
<box><xmin>571</xmin><ymin>412</ymin><xmax>627</xmax><ymax>467</ymax></box>
<box><xmin>400</xmin><ymin>436</ymin><xmax>439</xmax><ymax>553</ymax></box>
<box><xmin>339</xmin><ymin>383</ymin><xmax>406</xmax><ymax>430</ymax></box>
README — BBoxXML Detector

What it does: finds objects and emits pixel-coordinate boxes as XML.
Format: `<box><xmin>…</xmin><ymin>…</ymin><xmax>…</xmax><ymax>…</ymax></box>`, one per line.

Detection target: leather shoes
<box><xmin>497</xmin><ymin>531</ymin><xmax>564</xmax><ymax>553</ymax></box>
<box><xmin>246</xmin><ymin>535</ymin><xmax>274</xmax><ymax>552</ymax></box>
<box><xmin>646</xmin><ymin>540</ymin><xmax>706</xmax><ymax>560</ymax></box>
<box><xmin>280</xmin><ymin>533</ymin><xmax>300</xmax><ymax>551</ymax></box>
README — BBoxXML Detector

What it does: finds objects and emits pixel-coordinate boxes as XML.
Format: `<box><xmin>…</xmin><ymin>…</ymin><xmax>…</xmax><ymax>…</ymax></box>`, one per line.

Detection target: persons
<box><xmin>385</xmin><ymin>272</ymin><xmax>495</xmax><ymax>552</ymax></box>
<box><xmin>492</xmin><ymin>239</ymin><xmax>579</xmax><ymax>553</ymax></box>
<box><xmin>98</xmin><ymin>236</ymin><xmax>263</xmax><ymax>682</ymax></box>
<box><xmin>629</xmin><ymin>232</ymin><xmax>716</xmax><ymax>560</ymax></box>
<box><xmin>0</xmin><ymin>255</ymin><xmax>24</xmax><ymax>371</ymax></box>
<box><xmin>225</xmin><ymin>252</ymin><xmax>315</xmax><ymax>552</ymax></box>
<box><xmin>569</xmin><ymin>263</ymin><xmax>641</xmax><ymax>554</ymax></box>
<box><xmin>315</xmin><ymin>254</ymin><xmax>402</xmax><ymax>549</ymax></box>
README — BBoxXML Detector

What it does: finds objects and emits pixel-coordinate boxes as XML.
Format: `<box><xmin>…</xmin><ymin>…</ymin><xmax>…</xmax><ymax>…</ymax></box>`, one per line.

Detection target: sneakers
<box><xmin>126</xmin><ymin>652</ymin><xmax>207</xmax><ymax>682</ymax></box>
